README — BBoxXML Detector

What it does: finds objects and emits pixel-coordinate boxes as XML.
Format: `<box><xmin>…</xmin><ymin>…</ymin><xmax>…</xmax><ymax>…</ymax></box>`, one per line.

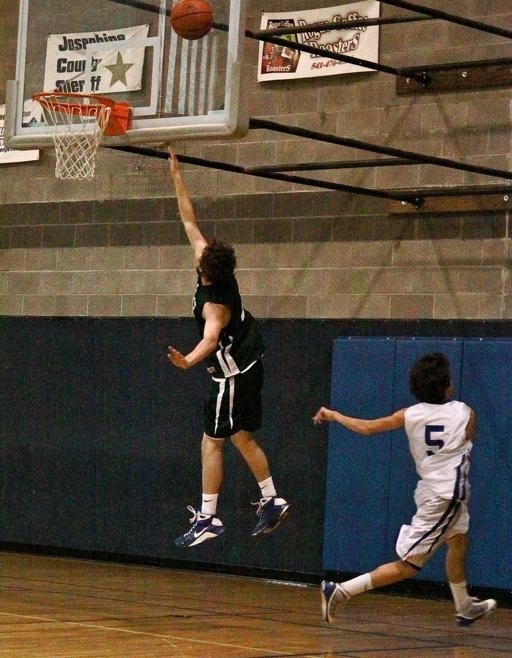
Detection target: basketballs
<box><xmin>171</xmin><ymin>0</ymin><xmax>212</xmax><ymax>40</ymax></box>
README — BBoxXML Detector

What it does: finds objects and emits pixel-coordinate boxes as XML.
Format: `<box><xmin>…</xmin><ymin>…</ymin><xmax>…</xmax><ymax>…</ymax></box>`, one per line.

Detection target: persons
<box><xmin>310</xmin><ymin>351</ymin><xmax>500</xmax><ymax>627</ymax></box>
<box><xmin>163</xmin><ymin>145</ymin><xmax>293</xmax><ymax>547</ymax></box>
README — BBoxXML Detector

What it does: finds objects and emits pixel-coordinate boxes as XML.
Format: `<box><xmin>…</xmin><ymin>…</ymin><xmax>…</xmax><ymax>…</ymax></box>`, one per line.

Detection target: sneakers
<box><xmin>456</xmin><ymin>596</ymin><xmax>497</xmax><ymax>627</ymax></box>
<box><xmin>250</xmin><ymin>496</ymin><xmax>292</xmax><ymax>536</ymax></box>
<box><xmin>321</xmin><ymin>580</ymin><xmax>350</xmax><ymax>624</ymax></box>
<box><xmin>174</xmin><ymin>513</ymin><xmax>224</xmax><ymax>549</ymax></box>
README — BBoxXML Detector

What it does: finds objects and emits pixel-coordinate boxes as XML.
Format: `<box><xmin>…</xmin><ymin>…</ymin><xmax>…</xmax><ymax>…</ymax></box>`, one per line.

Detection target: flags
<box><xmin>255</xmin><ymin>0</ymin><xmax>382</xmax><ymax>82</ymax></box>
